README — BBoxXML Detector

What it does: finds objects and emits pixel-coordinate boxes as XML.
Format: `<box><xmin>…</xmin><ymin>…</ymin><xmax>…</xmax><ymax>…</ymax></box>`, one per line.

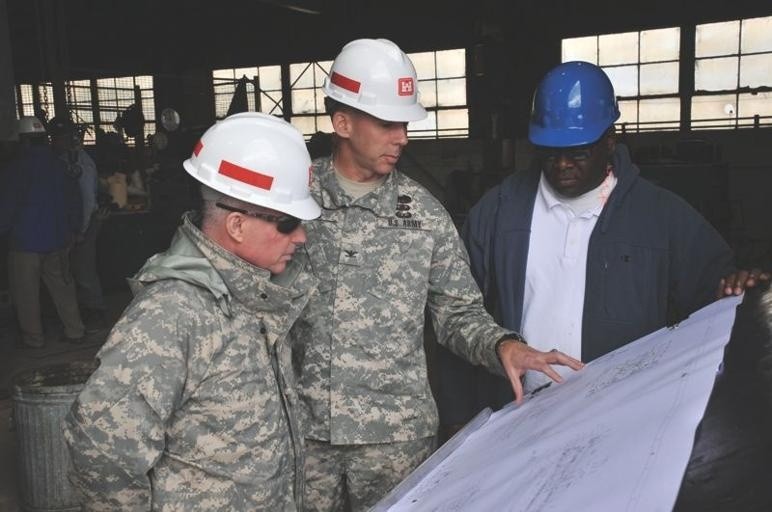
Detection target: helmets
<box><xmin>17</xmin><ymin>116</ymin><xmax>46</xmax><ymax>134</ymax></box>
<box><xmin>321</xmin><ymin>38</ymin><xmax>427</xmax><ymax>122</ymax></box>
<box><xmin>183</xmin><ymin>112</ymin><xmax>322</xmax><ymax>220</ymax></box>
<box><xmin>528</xmin><ymin>61</ymin><xmax>620</xmax><ymax>147</ymax></box>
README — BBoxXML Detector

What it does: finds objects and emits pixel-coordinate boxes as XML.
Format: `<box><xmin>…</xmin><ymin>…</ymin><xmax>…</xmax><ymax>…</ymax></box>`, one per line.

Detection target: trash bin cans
<box><xmin>7</xmin><ymin>361</ymin><xmax>96</xmax><ymax>512</ymax></box>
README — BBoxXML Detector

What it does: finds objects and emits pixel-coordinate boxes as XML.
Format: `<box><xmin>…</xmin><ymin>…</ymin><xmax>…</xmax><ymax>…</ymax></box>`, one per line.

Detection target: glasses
<box><xmin>215</xmin><ymin>203</ymin><xmax>300</xmax><ymax>233</ymax></box>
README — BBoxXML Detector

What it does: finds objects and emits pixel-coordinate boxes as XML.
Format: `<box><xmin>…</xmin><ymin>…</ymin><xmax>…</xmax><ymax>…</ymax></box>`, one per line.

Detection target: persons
<box><xmin>433</xmin><ymin>62</ymin><xmax>772</xmax><ymax>421</ymax></box>
<box><xmin>1</xmin><ymin>117</ymin><xmax>85</xmax><ymax>349</ymax></box>
<box><xmin>47</xmin><ymin>116</ymin><xmax>105</xmax><ymax>333</ymax></box>
<box><xmin>61</xmin><ymin>110</ymin><xmax>321</xmax><ymax>511</ymax></box>
<box><xmin>274</xmin><ymin>39</ymin><xmax>584</xmax><ymax>511</ymax></box>
